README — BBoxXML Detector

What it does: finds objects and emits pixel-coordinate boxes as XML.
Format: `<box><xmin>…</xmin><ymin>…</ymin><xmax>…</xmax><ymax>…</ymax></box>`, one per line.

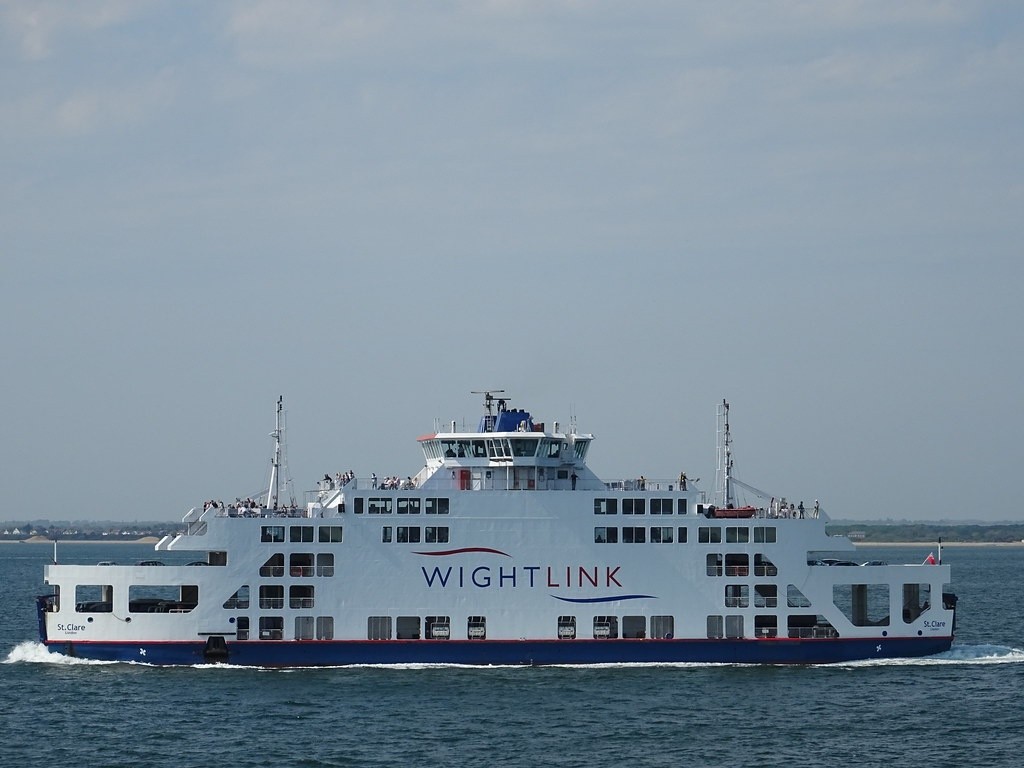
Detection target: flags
<box><xmin>927</xmin><ymin>555</ymin><xmax>936</xmax><ymax>564</ymax></box>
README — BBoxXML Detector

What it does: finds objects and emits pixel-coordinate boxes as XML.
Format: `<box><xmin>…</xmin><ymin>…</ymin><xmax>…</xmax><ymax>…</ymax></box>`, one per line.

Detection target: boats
<box><xmin>35</xmin><ymin>390</ymin><xmax>959</xmax><ymax>666</ymax></box>
<box><xmin>714</xmin><ymin>505</ymin><xmax>756</xmax><ymax>519</ymax></box>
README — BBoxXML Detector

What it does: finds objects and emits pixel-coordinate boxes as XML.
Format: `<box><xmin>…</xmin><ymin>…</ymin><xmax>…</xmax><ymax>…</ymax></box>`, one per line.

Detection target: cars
<box><xmin>865</xmin><ymin>560</ymin><xmax>889</xmax><ymax>566</ymax></box>
<box><xmin>821</xmin><ymin>559</ymin><xmax>839</xmax><ymax>566</ymax></box>
<box><xmin>807</xmin><ymin>560</ymin><xmax>829</xmax><ymax>566</ymax></box>
<box><xmin>832</xmin><ymin>561</ymin><xmax>860</xmax><ymax>566</ymax></box>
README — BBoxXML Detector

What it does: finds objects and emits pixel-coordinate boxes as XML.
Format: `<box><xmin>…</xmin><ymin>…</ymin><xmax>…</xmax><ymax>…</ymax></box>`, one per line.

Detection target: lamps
<box><xmin>452</xmin><ymin>470</ymin><xmax>456</xmax><ymax>480</ymax></box>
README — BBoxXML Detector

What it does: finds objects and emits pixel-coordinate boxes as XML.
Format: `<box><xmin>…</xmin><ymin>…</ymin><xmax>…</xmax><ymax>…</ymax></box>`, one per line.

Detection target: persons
<box><xmin>777</xmin><ymin>500</ymin><xmax>819</xmax><ymax>519</ymax></box>
<box><xmin>569</xmin><ymin>471</ymin><xmax>690</xmax><ymax>490</ymax></box>
<box><xmin>205</xmin><ymin>469</ymin><xmax>415</xmax><ymax>518</ymax></box>
